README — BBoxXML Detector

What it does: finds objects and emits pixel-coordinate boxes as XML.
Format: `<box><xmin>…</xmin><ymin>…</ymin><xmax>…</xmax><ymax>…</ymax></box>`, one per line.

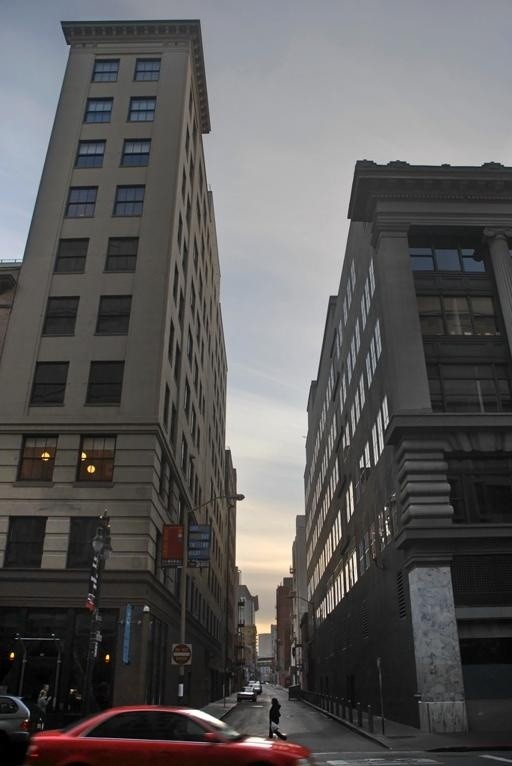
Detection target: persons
<box><xmin>268</xmin><ymin>698</ymin><xmax>286</xmax><ymax>741</ymax></box>
<box><xmin>37</xmin><ymin>684</ymin><xmax>52</xmax><ymax>710</ymax></box>
<box><xmin>38</xmin><ymin>690</ymin><xmax>49</xmax><ymax>731</ymax></box>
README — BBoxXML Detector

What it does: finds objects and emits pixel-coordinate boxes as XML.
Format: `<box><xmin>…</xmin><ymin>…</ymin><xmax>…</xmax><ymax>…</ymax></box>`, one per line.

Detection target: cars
<box><xmin>23</xmin><ymin>704</ymin><xmax>316</xmax><ymax>765</ymax></box>
<box><xmin>235</xmin><ymin>679</ymin><xmax>264</xmax><ymax>706</ymax></box>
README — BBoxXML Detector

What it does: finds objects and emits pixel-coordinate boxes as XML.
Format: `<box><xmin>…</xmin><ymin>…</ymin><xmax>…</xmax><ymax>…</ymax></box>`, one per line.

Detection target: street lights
<box><xmin>175</xmin><ymin>490</ymin><xmax>245</xmax><ymax>705</ymax></box>
<box><xmin>76</xmin><ymin>506</ymin><xmax>118</xmax><ymax>724</ymax></box>
<box><xmin>285</xmin><ymin>594</ymin><xmax>317</xmax><ymax>691</ymax></box>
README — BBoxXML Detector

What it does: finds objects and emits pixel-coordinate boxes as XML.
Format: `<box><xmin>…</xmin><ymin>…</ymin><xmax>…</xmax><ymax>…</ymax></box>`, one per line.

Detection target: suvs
<box><xmin>0</xmin><ymin>694</ymin><xmax>46</xmax><ymax>757</ymax></box>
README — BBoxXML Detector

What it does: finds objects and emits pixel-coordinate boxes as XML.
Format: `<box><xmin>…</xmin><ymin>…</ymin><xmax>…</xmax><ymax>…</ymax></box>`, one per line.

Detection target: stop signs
<box><xmin>172</xmin><ymin>644</ymin><xmax>193</xmax><ymax>666</ymax></box>
